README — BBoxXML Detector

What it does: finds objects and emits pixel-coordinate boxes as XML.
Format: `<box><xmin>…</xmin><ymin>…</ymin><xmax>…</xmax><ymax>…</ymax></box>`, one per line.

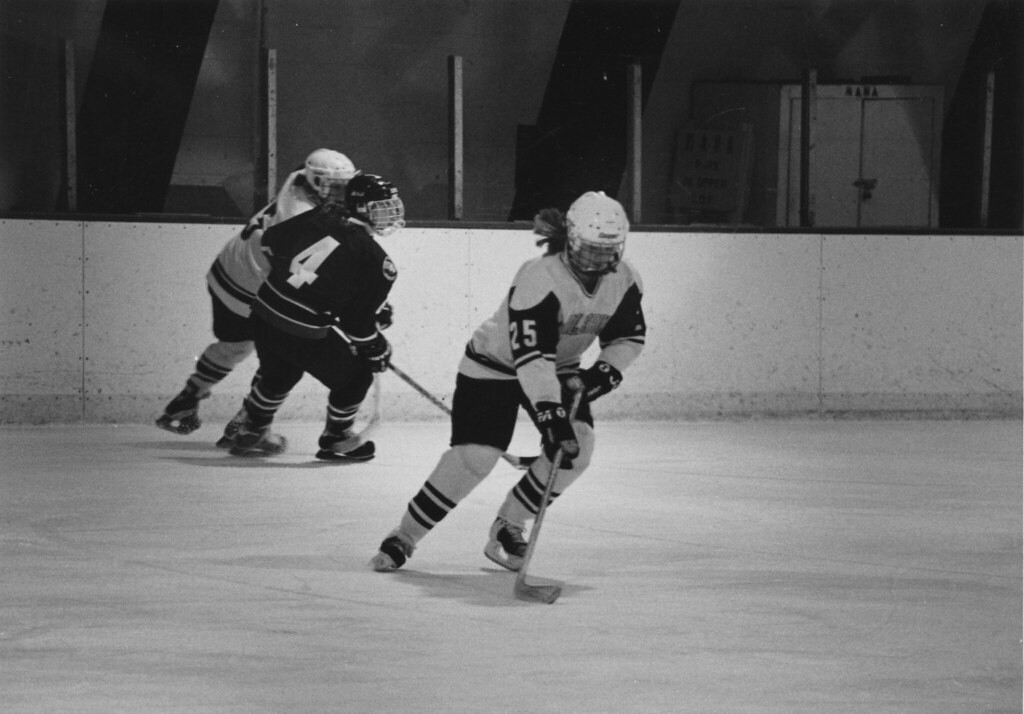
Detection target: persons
<box><xmin>229</xmin><ymin>172</ymin><xmax>393</xmax><ymax>460</ymax></box>
<box><xmin>370</xmin><ymin>190</ymin><xmax>646</xmax><ymax>572</ymax></box>
<box><xmin>152</xmin><ymin>148</ymin><xmax>357</xmax><ymax>434</ymax></box>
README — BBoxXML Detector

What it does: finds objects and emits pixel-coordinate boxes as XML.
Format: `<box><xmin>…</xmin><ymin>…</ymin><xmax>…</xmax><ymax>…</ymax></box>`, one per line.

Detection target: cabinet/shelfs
<box><xmin>693</xmin><ymin>77</ymin><xmax>944</xmax><ymax>231</ymax></box>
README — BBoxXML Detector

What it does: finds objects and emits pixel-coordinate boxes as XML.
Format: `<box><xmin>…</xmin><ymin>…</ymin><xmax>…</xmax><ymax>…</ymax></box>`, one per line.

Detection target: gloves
<box><xmin>534</xmin><ymin>402</ymin><xmax>581</xmax><ymax>471</ymax></box>
<box><xmin>379</xmin><ymin>302</ymin><xmax>395</xmax><ymax>327</ymax></box>
<box><xmin>356</xmin><ymin>334</ymin><xmax>392</xmax><ymax>373</ymax></box>
<box><xmin>562</xmin><ymin>361</ymin><xmax>624</xmax><ymax>405</ymax></box>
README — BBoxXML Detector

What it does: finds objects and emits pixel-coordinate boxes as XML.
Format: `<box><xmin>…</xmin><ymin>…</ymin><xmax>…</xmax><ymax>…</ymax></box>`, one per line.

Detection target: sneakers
<box><xmin>368</xmin><ymin>532</ymin><xmax>408</xmax><ymax>573</ymax></box>
<box><xmin>156</xmin><ymin>391</ymin><xmax>201</xmax><ymax>435</ymax></box>
<box><xmin>485</xmin><ymin>518</ymin><xmax>528</xmax><ymax>571</ymax></box>
<box><xmin>217</xmin><ymin>419</ymin><xmax>247</xmax><ymax>447</ymax></box>
<box><xmin>315</xmin><ymin>426</ymin><xmax>375</xmax><ymax>461</ymax></box>
<box><xmin>230</xmin><ymin>424</ymin><xmax>286</xmax><ymax>457</ymax></box>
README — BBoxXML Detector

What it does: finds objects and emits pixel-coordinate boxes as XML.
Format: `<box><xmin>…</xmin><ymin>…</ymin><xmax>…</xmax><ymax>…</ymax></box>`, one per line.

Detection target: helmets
<box><xmin>347</xmin><ymin>172</ymin><xmax>397</xmax><ymax>223</ymax></box>
<box><xmin>564</xmin><ymin>190</ymin><xmax>630</xmax><ymax>268</ymax></box>
<box><xmin>303</xmin><ymin>147</ymin><xmax>356</xmax><ymax>198</ymax></box>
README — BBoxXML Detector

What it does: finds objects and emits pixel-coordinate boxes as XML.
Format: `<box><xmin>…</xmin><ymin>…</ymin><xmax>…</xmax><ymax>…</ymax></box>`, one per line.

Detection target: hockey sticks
<box><xmin>333</xmin><ymin>319</ymin><xmax>540</xmax><ymax>470</ymax></box>
<box><xmin>513</xmin><ymin>389</ymin><xmax>582</xmax><ymax>605</ymax></box>
<box><xmin>375</xmin><ymin>302</ymin><xmax>393</xmax><ymax>329</ymax></box>
